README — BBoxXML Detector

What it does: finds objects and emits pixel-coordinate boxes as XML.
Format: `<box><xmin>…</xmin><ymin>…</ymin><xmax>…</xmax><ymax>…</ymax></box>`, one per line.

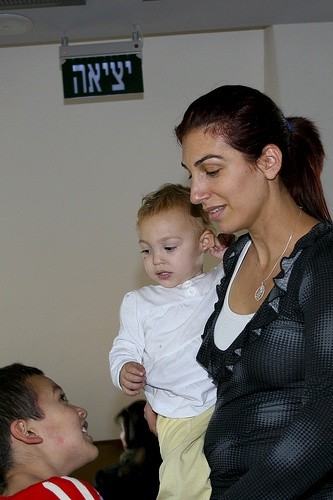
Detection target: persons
<box><xmin>95</xmin><ymin>399</ymin><xmax>163</xmax><ymax>500</ymax></box>
<box><xmin>0</xmin><ymin>363</ymin><xmax>105</xmax><ymax>500</ymax></box>
<box><xmin>109</xmin><ymin>183</ymin><xmax>239</xmax><ymax>500</ymax></box>
<box><xmin>145</xmin><ymin>84</ymin><xmax>333</xmax><ymax>500</ymax></box>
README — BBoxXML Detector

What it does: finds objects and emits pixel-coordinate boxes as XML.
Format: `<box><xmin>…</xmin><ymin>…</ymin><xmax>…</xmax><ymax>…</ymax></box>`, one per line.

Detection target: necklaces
<box><xmin>253</xmin><ymin>207</ymin><xmax>302</xmax><ymax>301</ymax></box>
